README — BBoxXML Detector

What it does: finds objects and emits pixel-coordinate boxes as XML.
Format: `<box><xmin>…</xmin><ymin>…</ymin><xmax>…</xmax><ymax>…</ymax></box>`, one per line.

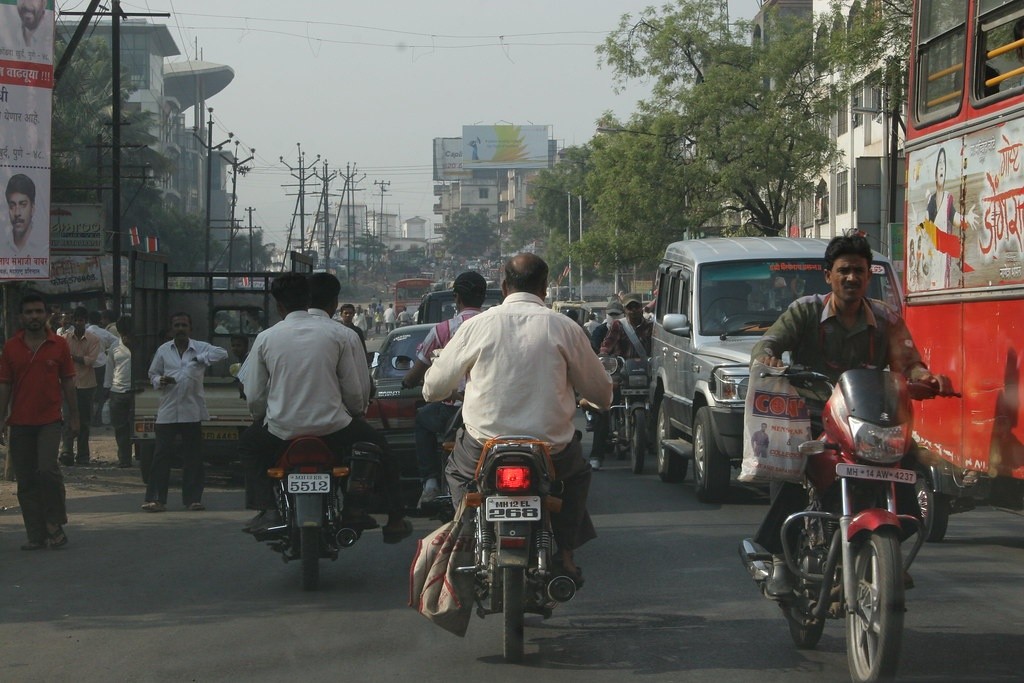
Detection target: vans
<box><xmin>646</xmin><ymin>238</ymin><xmax>904</xmax><ymax>506</ymax></box>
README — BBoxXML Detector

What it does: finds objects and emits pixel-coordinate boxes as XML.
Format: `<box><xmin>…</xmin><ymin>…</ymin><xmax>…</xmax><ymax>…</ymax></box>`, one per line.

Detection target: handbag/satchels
<box><xmin>736</xmin><ymin>361</ymin><xmax>814</xmax><ymax>487</ymax></box>
<box><xmin>406</xmin><ymin>503</ymin><xmax>475</xmax><ymax>639</ymax></box>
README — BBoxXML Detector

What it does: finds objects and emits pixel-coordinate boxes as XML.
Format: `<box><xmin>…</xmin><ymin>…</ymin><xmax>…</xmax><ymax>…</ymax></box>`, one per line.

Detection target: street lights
<box><xmin>852</xmin><ymin>107</ymin><xmax>890</xmax><ymax>156</ymax></box>
<box><xmin>597</xmin><ymin>128</ymin><xmax>690</xmax><ymax>240</ymax></box>
<box><xmin>537</xmin><ymin>185</ymin><xmax>584</xmax><ymax>302</ymax></box>
<box><xmin>522</xmin><ymin>183</ymin><xmax>572</xmax><ymax>301</ymax></box>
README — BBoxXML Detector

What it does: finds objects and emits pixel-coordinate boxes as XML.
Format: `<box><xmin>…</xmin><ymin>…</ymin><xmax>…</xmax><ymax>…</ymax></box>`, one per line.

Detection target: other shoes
<box><xmin>60</xmin><ymin>452</ymin><xmax>74</xmax><ymax>466</ymax></box>
<box><xmin>568</xmin><ymin>567</ymin><xmax>585</xmax><ymax>588</ymax></box>
<box><xmin>248</xmin><ymin>512</ymin><xmax>282</xmax><ymax>534</ymax></box>
<box><xmin>382</xmin><ymin>521</ymin><xmax>413</xmax><ymax>544</ymax></box>
<box><xmin>416</xmin><ymin>483</ymin><xmax>439</xmax><ymax>511</ymax></box>
<box><xmin>76</xmin><ymin>455</ymin><xmax>90</xmax><ymax>465</ymax></box>
<box><xmin>187</xmin><ymin>503</ymin><xmax>204</xmax><ymax>510</ymax></box>
<box><xmin>142</xmin><ymin>503</ymin><xmax>165</xmax><ymax>510</ymax></box>
<box><xmin>115</xmin><ymin>461</ymin><xmax>131</xmax><ymax>468</ymax></box>
<box><xmin>242</xmin><ymin>512</ymin><xmax>264</xmax><ymax>530</ymax></box>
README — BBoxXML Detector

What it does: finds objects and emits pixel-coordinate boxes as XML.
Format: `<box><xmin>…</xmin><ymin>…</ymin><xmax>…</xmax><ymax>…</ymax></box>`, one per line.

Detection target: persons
<box><xmin>421</xmin><ymin>251</ymin><xmax>613</xmax><ymax>591</ymax></box>
<box><xmin>16</xmin><ymin>0</ymin><xmax>49</xmax><ymax>47</ymax></box>
<box><xmin>0</xmin><ymin>294</ymin><xmax>79</xmax><ymax>552</ymax></box>
<box><xmin>4</xmin><ymin>174</ymin><xmax>36</xmax><ymax>251</ymax></box>
<box><xmin>909</xmin><ymin>235</ymin><xmax>927</xmax><ymax>291</ymax></box>
<box><xmin>141</xmin><ymin>312</ymin><xmax>228</xmax><ymax>513</ymax></box>
<box><xmin>925</xmin><ymin>147</ymin><xmax>979</xmax><ymax>236</ymax></box>
<box><xmin>751</xmin><ymin>422</ymin><xmax>769</xmax><ymax>458</ymax></box>
<box><xmin>401</xmin><ymin>272</ymin><xmax>487</xmax><ymax>509</ymax></box>
<box><xmin>749</xmin><ymin>228</ymin><xmax>955</xmax><ymax>596</ymax></box>
<box><xmin>47</xmin><ymin>295</ymin><xmax>654</xmax><ymax>471</ymax></box>
<box><xmin>237</xmin><ymin>271</ymin><xmax>413</xmax><ymax>544</ymax></box>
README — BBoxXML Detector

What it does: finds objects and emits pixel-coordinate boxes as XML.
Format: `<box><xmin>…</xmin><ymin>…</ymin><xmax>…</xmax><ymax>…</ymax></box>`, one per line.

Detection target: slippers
<box><xmin>48</xmin><ymin>522</ymin><xmax>67</xmax><ymax>548</ymax></box>
<box><xmin>20</xmin><ymin>539</ymin><xmax>42</xmax><ymax>552</ymax></box>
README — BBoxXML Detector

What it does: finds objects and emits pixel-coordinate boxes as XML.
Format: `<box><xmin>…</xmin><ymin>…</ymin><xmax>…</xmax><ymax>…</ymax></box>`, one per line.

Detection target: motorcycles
<box><xmin>584</xmin><ymin>353</ymin><xmax>652</xmax><ymax>476</ymax></box>
<box><xmin>738</xmin><ymin>369</ymin><xmax>962</xmax><ymax>683</ymax></box>
<box><xmin>453</xmin><ymin>357</ymin><xmax>618</xmax><ymax>662</ymax></box>
<box><xmin>391</xmin><ymin>355</ymin><xmax>466</xmax><ymax>523</ymax></box>
<box><xmin>228</xmin><ymin>362</ymin><xmax>384</xmax><ymax>591</ymax></box>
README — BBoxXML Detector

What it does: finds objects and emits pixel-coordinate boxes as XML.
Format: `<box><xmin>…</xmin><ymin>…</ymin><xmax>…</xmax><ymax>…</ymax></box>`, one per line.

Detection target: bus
<box><xmin>901</xmin><ymin>0</ymin><xmax>1024</xmax><ymax>544</ymax></box>
<box><xmin>393</xmin><ymin>279</ymin><xmax>436</xmax><ymax>318</ymax></box>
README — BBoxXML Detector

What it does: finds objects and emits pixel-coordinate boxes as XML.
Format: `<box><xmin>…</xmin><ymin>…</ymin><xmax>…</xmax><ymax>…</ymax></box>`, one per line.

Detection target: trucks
<box><xmin>127</xmin><ymin>250</ymin><xmax>313</xmax><ymax>484</ymax></box>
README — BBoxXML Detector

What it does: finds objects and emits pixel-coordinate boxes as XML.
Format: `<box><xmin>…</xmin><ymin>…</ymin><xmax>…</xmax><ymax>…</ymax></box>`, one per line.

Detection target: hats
<box><xmin>622</xmin><ymin>292</ymin><xmax>642</xmax><ymax>307</ymax></box>
<box><xmin>606</xmin><ymin>300</ymin><xmax>625</xmax><ymax>314</ymax></box>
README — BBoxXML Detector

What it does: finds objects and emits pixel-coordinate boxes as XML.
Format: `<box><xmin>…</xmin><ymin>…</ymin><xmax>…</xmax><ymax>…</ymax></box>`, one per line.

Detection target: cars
<box><xmin>363</xmin><ymin>322</ymin><xmax>464</xmax><ymax>476</ymax></box>
<box><xmin>417</xmin><ymin>289</ymin><xmax>505</xmax><ymax>325</ymax></box>
<box><xmin>551</xmin><ymin>301</ymin><xmax>607</xmax><ymax>325</ymax></box>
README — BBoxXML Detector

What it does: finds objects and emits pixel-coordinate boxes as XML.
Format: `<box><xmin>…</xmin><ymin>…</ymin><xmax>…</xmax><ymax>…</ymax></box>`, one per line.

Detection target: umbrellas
<box><xmin>50</xmin><ymin>209</ymin><xmax>72</xmax><ymax>226</ymax></box>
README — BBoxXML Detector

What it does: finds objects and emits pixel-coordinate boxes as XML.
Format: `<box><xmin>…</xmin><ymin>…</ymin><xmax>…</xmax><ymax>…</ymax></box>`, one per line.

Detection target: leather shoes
<box><xmin>763</xmin><ymin>564</ymin><xmax>795</xmax><ymax>599</ymax></box>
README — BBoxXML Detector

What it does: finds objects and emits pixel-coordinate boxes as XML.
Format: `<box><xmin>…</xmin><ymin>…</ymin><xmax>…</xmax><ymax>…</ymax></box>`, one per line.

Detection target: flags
<box><xmin>127</xmin><ymin>223</ymin><xmax>143</xmax><ymax>247</ymax></box>
<box><xmin>145</xmin><ymin>235</ymin><xmax>160</xmax><ymax>254</ymax></box>
